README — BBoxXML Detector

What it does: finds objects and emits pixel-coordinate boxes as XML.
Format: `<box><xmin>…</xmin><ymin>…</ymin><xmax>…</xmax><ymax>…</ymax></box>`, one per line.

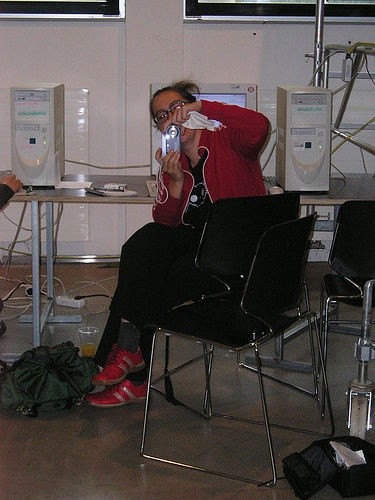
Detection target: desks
<box><xmin>1</xmin><ymin>173</ymin><xmax>375</xmax><ymax>364</ymax></box>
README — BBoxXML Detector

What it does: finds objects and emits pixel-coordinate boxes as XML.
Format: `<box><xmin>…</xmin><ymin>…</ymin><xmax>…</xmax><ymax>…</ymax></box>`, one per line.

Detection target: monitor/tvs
<box><xmin>148</xmin><ymin>82</ymin><xmax>256</xmax><ymax>175</ymax></box>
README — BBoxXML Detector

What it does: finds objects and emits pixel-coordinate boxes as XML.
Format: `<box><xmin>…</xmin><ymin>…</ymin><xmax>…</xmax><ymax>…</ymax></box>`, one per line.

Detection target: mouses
<box><xmin>268</xmin><ymin>186</ymin><xmax>283</xmax><ymax>195</ymax></box>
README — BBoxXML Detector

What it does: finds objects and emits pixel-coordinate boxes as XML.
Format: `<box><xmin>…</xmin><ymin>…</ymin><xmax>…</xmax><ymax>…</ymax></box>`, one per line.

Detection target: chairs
<box><xmin>141</xmin><ymin>190</ymin><xmax>375</xmax><ymax>486</ymax></box>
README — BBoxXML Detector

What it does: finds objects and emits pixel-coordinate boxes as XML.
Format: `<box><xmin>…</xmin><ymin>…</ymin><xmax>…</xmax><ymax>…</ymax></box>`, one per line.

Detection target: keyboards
<box><xmin>146</xmin><ymin>180</ymin><xmax>157</xmax><ymax>198</ymax></box>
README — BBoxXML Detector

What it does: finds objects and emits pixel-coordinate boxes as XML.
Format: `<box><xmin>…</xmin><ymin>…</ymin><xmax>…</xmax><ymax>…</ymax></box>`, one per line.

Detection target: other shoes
<box><xmin>85</xmin><ymin>378</ymin><xmax>150</xmax><ymax>409</ymax></box>
<box><xmin>91</xmin><ymin>342</ymin><xmax>145</xmax><ymax>385</ymax></box>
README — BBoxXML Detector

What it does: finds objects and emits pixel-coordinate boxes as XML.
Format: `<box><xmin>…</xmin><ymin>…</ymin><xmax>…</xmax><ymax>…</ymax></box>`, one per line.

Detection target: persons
<box><xmin>0</xmin><ymin>174</ymin><xmax>23</xmax><ymax>208</ymax></box>
<box><xmin>84</xmin><ymin>80</ymin><xmax>270</xmax><ymax>408</ymax></box>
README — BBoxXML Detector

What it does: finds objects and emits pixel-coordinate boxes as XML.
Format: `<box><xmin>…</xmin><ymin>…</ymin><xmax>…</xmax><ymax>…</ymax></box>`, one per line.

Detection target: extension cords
<box><xmin>56</xmin><ymin>296</ymin><xmax>85</xmax><ymax>308</ymax></box>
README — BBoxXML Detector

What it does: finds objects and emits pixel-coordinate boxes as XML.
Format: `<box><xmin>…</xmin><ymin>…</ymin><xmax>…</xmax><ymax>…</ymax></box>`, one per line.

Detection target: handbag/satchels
<box><xmin>281</xmin><ymin>436</ymin><xmax>375</xmax><ymax>497</ymax></box>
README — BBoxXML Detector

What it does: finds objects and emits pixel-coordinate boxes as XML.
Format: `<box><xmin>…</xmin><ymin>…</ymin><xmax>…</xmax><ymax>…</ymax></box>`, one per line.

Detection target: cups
<box><xmin>78</xmin><ymin>327</ymin><xmax>99</xmax><ymax>357</ymax></box>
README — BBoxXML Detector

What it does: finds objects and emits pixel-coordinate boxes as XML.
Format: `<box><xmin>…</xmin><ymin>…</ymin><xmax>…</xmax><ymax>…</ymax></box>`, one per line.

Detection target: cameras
<box><xmin>161</xmin><ymin>124</ymin><xmax>180</xmax><ymax>158</ymax></box>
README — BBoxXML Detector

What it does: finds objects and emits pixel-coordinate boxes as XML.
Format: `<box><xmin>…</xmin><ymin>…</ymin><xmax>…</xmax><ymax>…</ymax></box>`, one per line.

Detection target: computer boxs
<box><xmin>275</xmin><ymin>86</ymin><xmax>331</xmax><ymax>195</ymax></box>
<box><xmin>10</xmin><ymin>83</ymin><xmax>64</xmax><ymax>186</ymax></box>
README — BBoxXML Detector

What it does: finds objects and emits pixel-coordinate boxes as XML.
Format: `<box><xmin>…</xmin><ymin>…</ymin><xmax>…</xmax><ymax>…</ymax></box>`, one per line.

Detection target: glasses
<box><xmin>152</xmin><ymin>101</ymin><xmax>194</xmax><ymax>124</ymax></box>
